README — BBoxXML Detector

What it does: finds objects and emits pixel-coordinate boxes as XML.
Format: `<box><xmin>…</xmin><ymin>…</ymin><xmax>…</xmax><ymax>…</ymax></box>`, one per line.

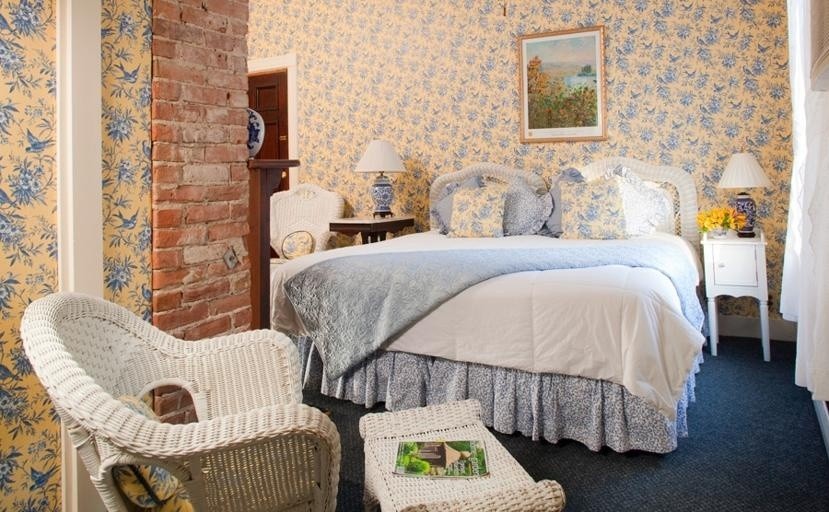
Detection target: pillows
<box><xmin>427</xmin><ymin>166</ymin><xmax>674</xmax><ymax>240</ymax></box>
<box><xmin>108</xmin><ymin>396</ymin><xmax>194</xmax><ymax>512</ymax></box>
<box><xmin>281</xmin><ymin>230</ymin><xmax>313</xmax><ymax>260</ymax></box>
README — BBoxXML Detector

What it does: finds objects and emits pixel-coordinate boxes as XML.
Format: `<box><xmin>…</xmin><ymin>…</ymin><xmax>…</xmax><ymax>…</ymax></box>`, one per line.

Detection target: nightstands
<box><xmin>330</xmin><ymin>216</ymin><xmax>414</xmax><ymax>244</ymax></box>
<box><xmin>700</xmin><ymin>228</ymin><xmax>772</xmax><ymax>362</ymax></box>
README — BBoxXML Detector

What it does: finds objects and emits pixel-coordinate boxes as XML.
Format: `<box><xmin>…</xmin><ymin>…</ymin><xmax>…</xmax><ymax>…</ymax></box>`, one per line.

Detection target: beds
<box><xmin>269</xmin><ymin>157</ymin><xmax>705</xmax><ymax>453</ymax></box>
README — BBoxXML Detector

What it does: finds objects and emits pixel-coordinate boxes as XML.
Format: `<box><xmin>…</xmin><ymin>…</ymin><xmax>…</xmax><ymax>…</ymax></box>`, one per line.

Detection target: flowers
<box><xmin>696</xmin><ymin>205</ymin><xmax>746</xmax><ymax>231</ymax></box>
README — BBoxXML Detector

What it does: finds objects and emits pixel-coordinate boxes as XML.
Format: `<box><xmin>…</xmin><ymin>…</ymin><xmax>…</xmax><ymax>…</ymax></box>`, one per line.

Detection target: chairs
<box><xmin>21</xmin><ymin>292</ymin><xmax>341</xmax><ymax>512</ymax></box>
<box><xmin>270</xmin><ymin>184</ymin><xmax>345</xmax><ymax>269</ymax></box>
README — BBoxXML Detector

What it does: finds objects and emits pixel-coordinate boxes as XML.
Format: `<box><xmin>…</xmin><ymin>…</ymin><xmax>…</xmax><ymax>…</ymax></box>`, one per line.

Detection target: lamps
<box><xmin>354</xmin><ymin>139</ymin><xmax>406</xmax><ymax>217</ymax></box>
<box><xmin>718</xmin><ymin>153</ymin><xmax>774</xmax><ymax>237</ymax></box>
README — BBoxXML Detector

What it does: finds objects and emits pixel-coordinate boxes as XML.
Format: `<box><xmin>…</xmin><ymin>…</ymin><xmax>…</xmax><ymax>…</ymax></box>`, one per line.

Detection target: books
<box><xmin>393</xmin><ymin>439</ymin><xmax>488</xmax><ymax>479</ymax></box>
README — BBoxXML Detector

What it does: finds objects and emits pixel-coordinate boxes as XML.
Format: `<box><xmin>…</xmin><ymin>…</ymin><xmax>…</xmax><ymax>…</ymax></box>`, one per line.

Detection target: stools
<box><xmin>359</xmin><ymin>398</ymin><xmax>566</xmax><ymax>511</ymax></box>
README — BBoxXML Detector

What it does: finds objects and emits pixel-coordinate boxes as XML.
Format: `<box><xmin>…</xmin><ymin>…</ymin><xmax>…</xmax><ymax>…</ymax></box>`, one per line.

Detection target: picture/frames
<box><xmin>518</xmin><ymin>25</ymin><xmax>608</xmax><ymax>142</ymax></box>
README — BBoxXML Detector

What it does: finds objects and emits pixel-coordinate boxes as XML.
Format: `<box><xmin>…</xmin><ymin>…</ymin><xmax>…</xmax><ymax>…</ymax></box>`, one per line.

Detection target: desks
<box><xmin>247</xmin><ymin>160</ymin><xmax>300</xmax><ymax>330</ymax></box>
